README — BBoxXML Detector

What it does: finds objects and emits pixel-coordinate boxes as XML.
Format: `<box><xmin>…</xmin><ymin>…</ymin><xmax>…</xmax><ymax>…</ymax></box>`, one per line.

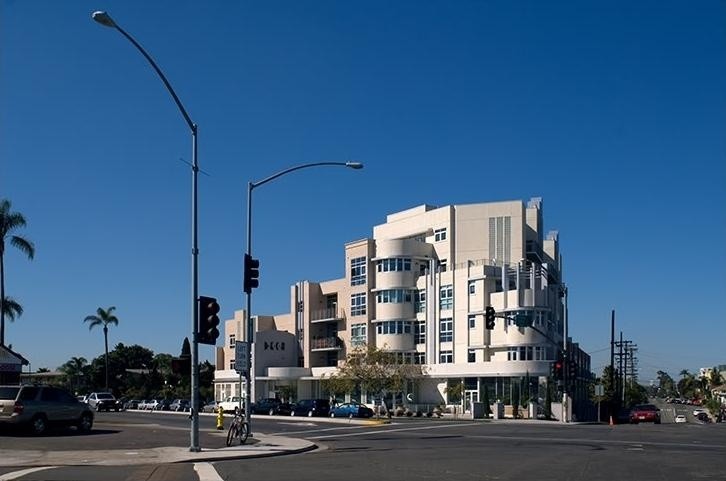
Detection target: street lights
<box><xmin>519</xmin><ymin>255</ymin><xmax>570</xmax><ymax>422</ymax></box>
<box><xmin>91</xmin><ymin>7</ymin><xmax>221</xmax><ymax>453</ymax></box>
<box><xmin>244</xmin><ymin>159</ymin><xmax>369</xmax><ymax>441</ymax></box>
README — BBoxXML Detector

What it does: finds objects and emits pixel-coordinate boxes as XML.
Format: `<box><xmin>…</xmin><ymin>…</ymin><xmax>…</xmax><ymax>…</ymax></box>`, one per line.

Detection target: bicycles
<box><xmin>225</xmin><ymin>406</ymin><xmax>248</xmax><ymax>448</ymax></box>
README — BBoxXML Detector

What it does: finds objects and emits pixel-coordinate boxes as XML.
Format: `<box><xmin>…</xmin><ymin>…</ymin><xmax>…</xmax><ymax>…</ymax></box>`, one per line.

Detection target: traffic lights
<box><xmin>243</xmin><ymin>253</ymin><xmax>260</xmax><ymax>294</ymax></box>
<box><xmin>567</xmin><ymin>361</ymin><xmax>577</xmax><ymax>380</ymax></box>
<box><xmin>485</xmin><ymin>306</ymin><xmax>496</xmax><ymax>330</ymax></box>
<box><xmin>196</xmin><ymin>296</ymin><xmax>221</xmax><ymax>348</ymax></box>
<box><xmin>555</xmin><ymin>362</ymin><xmax>563</xmax><ymax>380</ymax></box>
<box><xmin>549</xmin><ymin>361</ymin><xmax>556</xmax><ymax>379</ymax></box>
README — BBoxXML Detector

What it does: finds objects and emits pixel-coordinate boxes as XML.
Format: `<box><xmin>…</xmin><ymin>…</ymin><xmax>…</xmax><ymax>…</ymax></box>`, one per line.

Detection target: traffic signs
<box><xmin>236</xmin><ymin>340</ymin><xmax>250</xmax><ymax>374</ymax></box>
<box><xmin>514</xmin><ymin>315</ymin><xmax>533</xmax><ymax>328</ymax></box>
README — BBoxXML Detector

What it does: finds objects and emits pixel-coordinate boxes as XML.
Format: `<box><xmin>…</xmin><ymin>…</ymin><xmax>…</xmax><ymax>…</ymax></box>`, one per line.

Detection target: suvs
<box><xmin>0</xmin><ymin>386</ymin><xmax>95</xmax><ymax>436</ymax></box>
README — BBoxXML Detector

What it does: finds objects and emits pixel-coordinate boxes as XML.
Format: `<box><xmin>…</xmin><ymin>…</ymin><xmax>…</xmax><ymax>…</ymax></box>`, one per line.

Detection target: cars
<box><xmin>327</xmin><ymin>403</ymin><xmax>373</xmax><ymax>420</ymax></box>
<box><xmin>76</xmin><ymin>395</ymin><xmax>87</xmax><ymax>404</ymax></box>
<box><xmin>246</xmin><ymin>398</ymin><xmax>282</xmax><ymax>417</ymax></box>
<box><xmin>664</xmin><ymin>395</ymin><xmax>726</xmax><ymax>425</ymax></box>
<box><xmin>629</xmin><ymin>404</ymin><xmax>662</xmax><ymax>427</ymax></box>
<box><xmin>200</xmin><ymin>401</ymin><xmax>221</xmax><ymax>414</ymax></box>
<box><xmin>87</xmin><ymin>392</ymin><xmax>120</xmax><ymax>414</ymax></box>
<box><xmin>117</xmin><ymin>395</ymin><xmax>191</xmax><ymax>415</ymax></box>
<box><xmin>288</xmin><ymin>399</ymin><xmax>330</xmax><ymax>419</ymax></box>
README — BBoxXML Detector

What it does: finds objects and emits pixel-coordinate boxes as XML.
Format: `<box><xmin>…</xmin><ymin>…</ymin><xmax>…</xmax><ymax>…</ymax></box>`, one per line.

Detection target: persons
<box><xmin>329</xmin><ymin>396</ymin><xmax>339</xmax><ymax>408</ymax></box>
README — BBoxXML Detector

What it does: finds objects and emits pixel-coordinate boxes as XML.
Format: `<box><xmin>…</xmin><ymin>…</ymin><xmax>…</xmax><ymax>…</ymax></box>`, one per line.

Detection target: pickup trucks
<box><xmin>218</xmin><ymin>396</ymin><xmax>247</xmax><ymax>416</ymax></box>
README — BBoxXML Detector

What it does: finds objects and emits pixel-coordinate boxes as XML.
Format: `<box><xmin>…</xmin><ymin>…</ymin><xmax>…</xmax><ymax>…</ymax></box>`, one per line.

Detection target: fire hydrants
<box><xmin>215</xmin><ymin>406</ymin><xmax>224</xmax><ymax>430</ymax></box>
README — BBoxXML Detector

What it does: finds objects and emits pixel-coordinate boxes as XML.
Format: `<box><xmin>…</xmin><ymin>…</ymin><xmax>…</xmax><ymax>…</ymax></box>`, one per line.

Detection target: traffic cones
<box><xmin>608</xmin><ymin>416</ymin><xmax>614</xmax><ymax>427</ymax></box>
